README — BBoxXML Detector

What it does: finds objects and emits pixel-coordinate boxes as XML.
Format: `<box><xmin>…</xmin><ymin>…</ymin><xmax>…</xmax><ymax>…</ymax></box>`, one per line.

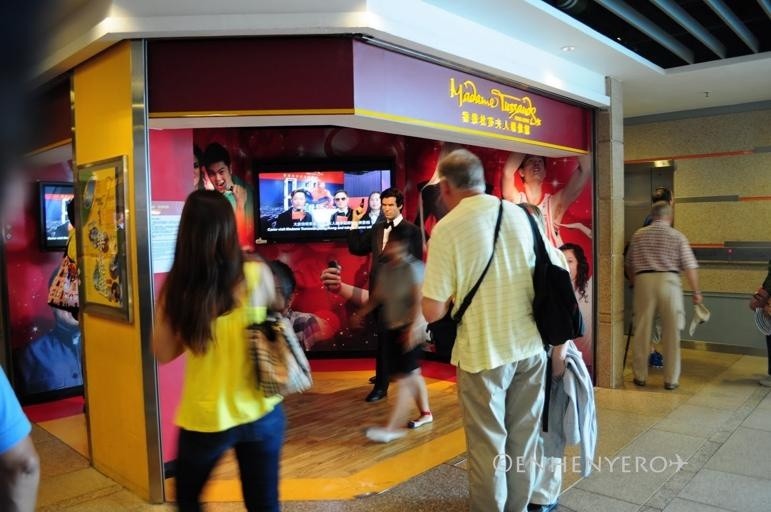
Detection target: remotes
<box><xmin>328</xmin><ymin>260</ymin><xmax>338</xmax><ymax>275</ymax></box>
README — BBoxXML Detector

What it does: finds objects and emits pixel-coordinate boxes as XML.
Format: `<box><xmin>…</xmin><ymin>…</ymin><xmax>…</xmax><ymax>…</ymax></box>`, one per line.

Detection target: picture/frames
<box><xmin>74</xmin><ymin>151</ymin><xmax>135</xmax><ymax>329</ymax></box>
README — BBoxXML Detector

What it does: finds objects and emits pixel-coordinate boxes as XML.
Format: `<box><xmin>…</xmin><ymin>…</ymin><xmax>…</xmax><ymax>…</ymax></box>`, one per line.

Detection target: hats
<box><xmin>689</xmin><ymin>300</ymin><xmax>711</xmax><ymax>335</ymax></box>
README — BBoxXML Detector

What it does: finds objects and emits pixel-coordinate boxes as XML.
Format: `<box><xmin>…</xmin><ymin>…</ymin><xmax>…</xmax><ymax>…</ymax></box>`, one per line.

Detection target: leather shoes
<box><xmin>364</xmin><ymin>426</ymin><xmax>407</xmax><ymax>444</ymax></box>
<box><xmin>365</xmin><ymin>374</ymin><xmax>388</xmax><ymax>402</ymax></box>
<box><xmin>407</xmin><ymin>412</ymin><xmax>434</xmax><ymax>428</ymax></box>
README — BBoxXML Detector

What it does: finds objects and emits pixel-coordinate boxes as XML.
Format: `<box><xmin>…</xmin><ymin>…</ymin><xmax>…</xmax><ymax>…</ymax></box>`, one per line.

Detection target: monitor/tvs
<box><xmin>37</xmin><ymin>181</ymin><xmax>75</xmax><ymax>251</ymax></box>
<box><xmin>255</xmin><ymin>155</ymin><xmax>396</xmax><ymax>246</ymax></box>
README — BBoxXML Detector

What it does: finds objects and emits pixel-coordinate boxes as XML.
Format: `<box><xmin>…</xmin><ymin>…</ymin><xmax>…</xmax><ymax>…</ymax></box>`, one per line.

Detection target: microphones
<box><xmin>224</xmin><ymin>183</ymin><xmax>233</xmax><ymax>191</ymax></box>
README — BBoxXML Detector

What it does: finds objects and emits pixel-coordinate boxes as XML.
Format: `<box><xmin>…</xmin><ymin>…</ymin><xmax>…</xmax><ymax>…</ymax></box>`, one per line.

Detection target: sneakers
<box><xmin>527</xmin><ymin>500</ymin><xmax>560</xmax><ymax>512</ymax></box>
<box><xmin>649</xmin><ymin>350</ymin><xmax>664</xmax><ymax>368</ymax></box>
<box><xmin>759</xmin><ymin>374</ymin><xmax>771</xmax><ymax>387</ymax></box>
<box><xmin>664</xmin><ymin>380</ymin><xmax>680</xmax><ymax>390</ymax></box>
<box><xmin>633</xmin><ymin>377</ymin><xmax>646</xmax><ymax>385</ymax></box>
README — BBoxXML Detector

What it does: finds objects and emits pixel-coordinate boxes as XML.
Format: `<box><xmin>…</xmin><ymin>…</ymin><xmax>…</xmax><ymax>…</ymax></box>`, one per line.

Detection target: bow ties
<box><xmin>385</xmin><ymin>221</ymin><xmax>393</xmax><ymax>229</ymax></box>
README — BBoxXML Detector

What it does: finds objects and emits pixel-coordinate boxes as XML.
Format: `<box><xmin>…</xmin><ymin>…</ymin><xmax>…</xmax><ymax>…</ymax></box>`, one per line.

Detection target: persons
<box><xmin>202</xmin><ymin>141</ymin><xmax>255</xmax><ymax>252</ymax></box>
<box><xmin>152</xmin><ymin>188</ymin><xmax>286</xmax><ymax>510</ymax></box>
<box><xmin>348</xmin><ymin>188</ymin><xmax>423</xmax><ymax>402</ymax></box>
<box><xmin>517</xmin><ymin>203</ymin><xmax>575</xmax><ymax>512</ymax></box>
<box><xmin>192</xmin><ymin>143</ymin><xmax>205</xmax><ymax>191</ymax></box>
<box><xmin>360</xmin><ymin>191</ymin><xmax>382</xmax><ymax>224</ymax></box>
<box><xmin>0</xmin><ymin>362</ymin><xmax>41</xmax><ymax>512</ymax></box>
<box><xmin>22</xmin><ymin>265</ymin><xmax>83</xmax><ymax>395</ymax></box>
<box><xmin>553</xmin><ymin>219</ymin><xmax>593</xmax><ymax>384</ymax></box>
<box><xmin>266</xmin><ymin>259</ymin><xmax>326</xmax><ymax>351</ymax></box>
<box><xmin>330</xmin><ymin>190</ymin><xmax>357</xmax><ymax>225</ymax></box>
<box><xmin>642</xmin><ymin>187</ymin><xmax>674</xmax><ymax>369</ymax></box>
<box><xmin>350</xmin><ymin>239</ymin><xmax>435</xmax><ymax>443</ymax></box>
<box><xmin>421</xmin><ymin>150</ymin><xmax>564</xmax><ymax>512</ymax></box>
<box><xmin>501</xmin><ymin>152</ymin><xmax>592</xmax><ymax>248</ymax></box>
<box><xmin>751</xmin><ymin>259</ymin><xmax>771</xmax><ymax>387</ymax></box>
<box><xmin>276</xmin><ymin>188</ymin><xmax>312</xmax><ymax>228</ymax></box>
<box><xmin>65</xmin><ymin>198</ymin><xmax>80</xmax><ymax>321</ymax></box>
<box><xmin>625</xmin><ymin>202</ymin><xmax>703</xmax><ymax>390</ymax></box>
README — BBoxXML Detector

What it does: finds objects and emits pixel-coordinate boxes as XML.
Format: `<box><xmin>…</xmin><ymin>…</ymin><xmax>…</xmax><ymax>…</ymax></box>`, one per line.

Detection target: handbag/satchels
<box><xmin>427</xmin><ymin>315</ymin><xmax>457</xmax><ymax>362</ymax></box>
<box><xmin>532</xmin><ymin>264</ymin><xmax>587</xmax><ymax>346</ymax></box>
<box><xmin>526</xmin><ymin>455</ymin><xmax>563</xmax><ymax>506</ymax></box>
<box><xmin>245</xmin><ymin>317</ymin><xmax>313</xmax><ymax>397</ymax></box>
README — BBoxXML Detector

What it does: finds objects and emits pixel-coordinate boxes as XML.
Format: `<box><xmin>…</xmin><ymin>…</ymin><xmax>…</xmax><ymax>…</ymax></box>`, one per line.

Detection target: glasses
<box><xmin>333</xmin><ymin>197</ymin><xmax>346</xmax><ymax>202</ymax></box>
